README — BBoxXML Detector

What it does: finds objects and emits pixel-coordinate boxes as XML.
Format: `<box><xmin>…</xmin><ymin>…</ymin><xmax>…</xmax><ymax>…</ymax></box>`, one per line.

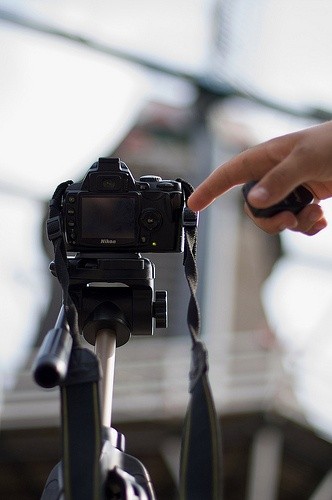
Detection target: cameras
<box><xmin>32</xmin><ymin>156</ymin><xmax>184</xmax><ymax>390</ymax></box>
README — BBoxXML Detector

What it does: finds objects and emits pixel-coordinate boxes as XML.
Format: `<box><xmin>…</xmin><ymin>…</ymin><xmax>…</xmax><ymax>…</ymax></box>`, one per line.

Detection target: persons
<box><xmin>186</xmin><ymin>117</ymin><xmax>332</xmax><ymax>237</ymax></box>
